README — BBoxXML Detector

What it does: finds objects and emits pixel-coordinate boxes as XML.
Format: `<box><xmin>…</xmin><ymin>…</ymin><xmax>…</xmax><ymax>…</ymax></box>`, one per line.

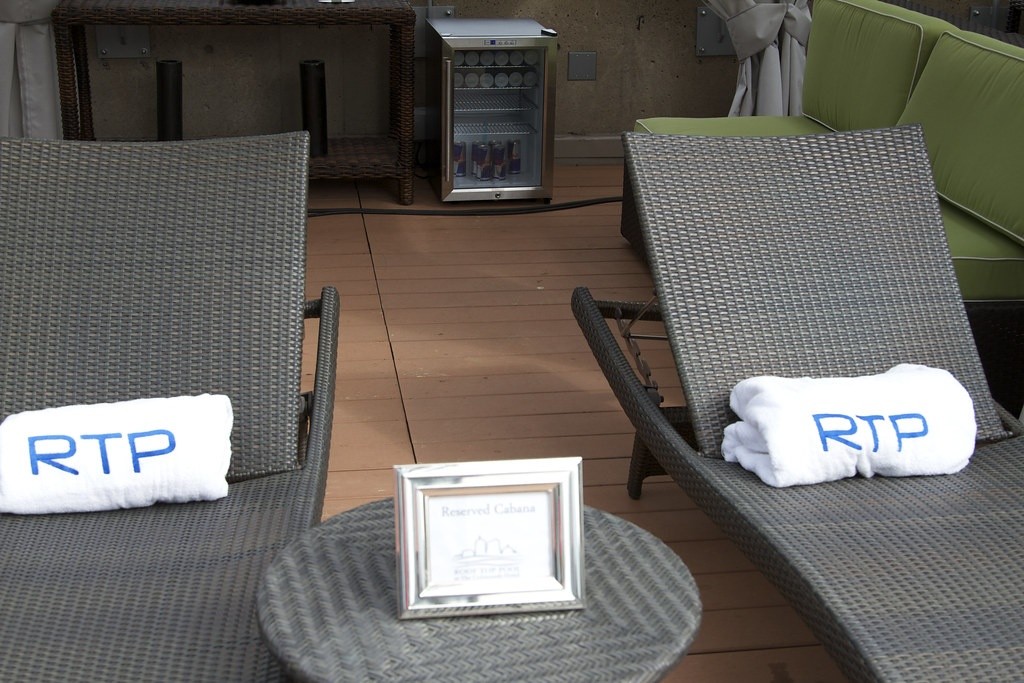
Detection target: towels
<box><xmin>0</xmin><ymin>392</ymin><xmax>234</xmax><ymax>516</ymax></box>
<box><xmin>721</xmin><ymin>363</ymin><xmax>976</xmax><ymax>489</ymax></box>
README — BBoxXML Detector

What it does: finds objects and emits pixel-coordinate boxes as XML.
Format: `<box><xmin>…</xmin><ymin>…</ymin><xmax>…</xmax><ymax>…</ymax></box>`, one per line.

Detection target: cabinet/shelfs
<box><xmin>49</xmin><ymin>0</ymin><xmax>417</xmax><ymax>209</ymax></box>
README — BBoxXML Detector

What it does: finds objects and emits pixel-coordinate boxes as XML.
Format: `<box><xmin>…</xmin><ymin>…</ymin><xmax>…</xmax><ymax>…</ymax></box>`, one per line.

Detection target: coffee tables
<box><xmin>254</xmin><ymin>499</ymin><xmax>706</xmax><ymax>683</ymax></box>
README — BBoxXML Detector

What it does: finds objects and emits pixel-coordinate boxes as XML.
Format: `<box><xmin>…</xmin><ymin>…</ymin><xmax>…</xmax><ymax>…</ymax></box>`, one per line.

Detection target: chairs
<box><xmin>569</xmin><ymin>132</ymin><xmax>1023</xmax><ymax>683</ymax></box>
<box><xmin>1</xmin><ymin>129</ymin><xmax>341</xmax><ymax>683</ymax></box>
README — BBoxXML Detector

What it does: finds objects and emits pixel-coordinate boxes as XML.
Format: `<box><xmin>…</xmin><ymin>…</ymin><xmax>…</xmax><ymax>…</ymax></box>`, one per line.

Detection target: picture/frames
<box><xmin>391</xmin><ymin>457</ymin><xmax>584</xmax><ymax>619</ymax></box>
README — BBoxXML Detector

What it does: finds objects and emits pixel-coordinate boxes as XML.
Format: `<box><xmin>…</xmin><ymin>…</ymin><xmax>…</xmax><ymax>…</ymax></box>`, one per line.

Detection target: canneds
<box><xmin>454</xmin><ymin>139</ymin><xmax>522</xmax><ymax>181</ymax></box>
<box><xmin>453</xmin><ymin>50</ymin><xmax>540</xmax><ymax>88</ymax></box>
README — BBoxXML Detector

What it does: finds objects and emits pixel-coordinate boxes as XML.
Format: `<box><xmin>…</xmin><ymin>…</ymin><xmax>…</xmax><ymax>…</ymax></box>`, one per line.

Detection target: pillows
<box><xmin>800</xmin><ymin>0</ymin><xmax>960</xmax><ymax>152</ymax></box>
<box><xmin>892</xmin><ymin>27</ymin><xmax>1024</xmax><ymax>247</ymax></box>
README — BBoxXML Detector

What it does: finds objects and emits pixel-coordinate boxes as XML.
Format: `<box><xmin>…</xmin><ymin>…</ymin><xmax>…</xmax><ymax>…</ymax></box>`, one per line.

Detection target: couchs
<box><xmin>620</xmin><ymin>0</ymin><xmax>1024</xmax><ymax>340</ymax></box>
